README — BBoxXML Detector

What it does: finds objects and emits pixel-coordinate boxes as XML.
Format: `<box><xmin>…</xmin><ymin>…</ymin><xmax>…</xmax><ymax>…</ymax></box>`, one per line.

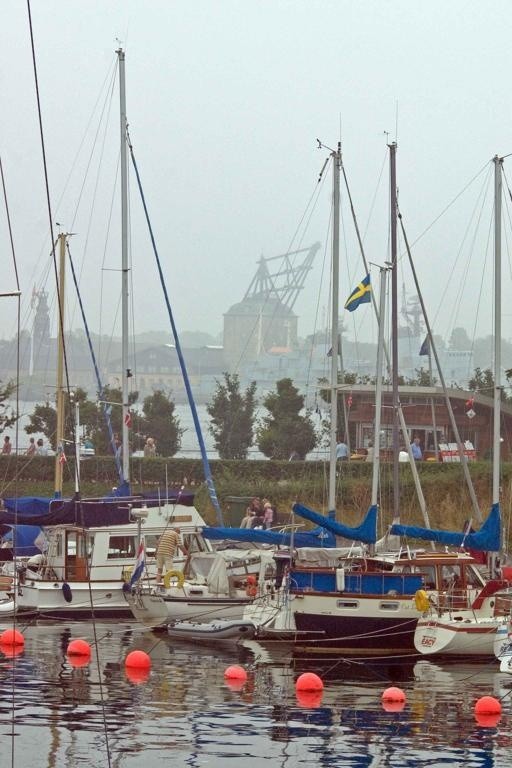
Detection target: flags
<box><xmin>419</xmin><ymin>336</ymin><xmax>429</xmax><ymax>355</ymax></box>
<box><xmin>464</xmin><ymin>396</ymin><xmax>474</xmax><ymax>410</ymax></box>
<box><xmin>343</xmin><ymin>394</ymin><xmax>353</xmax><ymax>407</ymax></box>
<box><xmin>343</xmin><ymin>273</ymin><xmax>371</xmax><ymax>313</ymax></box>
<box><xmin>326</xmin><ymin>336</ymin><xmax>342</xmax><ymax>358</ymax></box>
<box><xmin>124</xmin><ymin>408</ymin><xmax>133</xmax><ymax>428</ymax></box>
<box><xmin>121</xmin><ymin>542</ymin><xmax>146</xmax><ymax>591</ymax></box>
<box><xmin>58</xmin><ymin>442</ymin><xmax>69</xmax><ymax>464</ymax></box>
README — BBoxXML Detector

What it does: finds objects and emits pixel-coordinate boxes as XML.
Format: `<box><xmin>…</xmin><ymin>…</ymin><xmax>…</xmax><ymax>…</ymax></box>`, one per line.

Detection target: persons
<box><xmin>153</xmin><ymin>525</ymin><xmax>192</xmax><ymax>586</ymax></box>
<box><xmin>35</xmin><ymin>438</ymin><xmax>49</xmax><ymax>457</ymax></box>
<box><xmin>28</xmin><ymin>554</ymin><xmax>46</xmax><ymax>572</ymax></box>
<box><xmin>1</xmin><ymin>435</ymin><xmax>12</xmax><ymax>455</ymax></box>
<box><xmin>408</xmin><ymin>437</ymin><xmax>423</xmax><ymax>461</ymax></box>
<box><xmin>143</xmin><ymin>437</ymin><xmax>157</xmax><ymax>458</ymax></box>
<box><xmin>24</xmin><ymin>437</ymin><xmax>36</xmax><ymax>455</ymax></box>
<box><xmin>108</xmin><ymin>433</ymin><xmax>132</xmax><ymax>457</ymax></box>
<box><xmin>363</xmin><ymin>441</ymin><xmax>375</xmax><ymax>462</ymax></box>
<box><xmin>238</xmin><ymin>495</ymin><xmax>278</xmax><ymax>533</ymax></box>
<box><xmin>335</xmin><ymin>439</ymin><xmax>349</xmax><ymax>460</ymax></box>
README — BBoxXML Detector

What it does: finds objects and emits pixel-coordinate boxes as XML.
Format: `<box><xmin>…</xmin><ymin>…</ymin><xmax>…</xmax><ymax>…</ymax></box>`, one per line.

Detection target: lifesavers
<box><xmin>507</xmin><ymin>615</ymin><xmax>512</xmax><ymax>641</ymax></box>
<box><xmin>415</xmin><ymin>590</ymin><xmax>428</xmax><ymax>611</ymax></box>
